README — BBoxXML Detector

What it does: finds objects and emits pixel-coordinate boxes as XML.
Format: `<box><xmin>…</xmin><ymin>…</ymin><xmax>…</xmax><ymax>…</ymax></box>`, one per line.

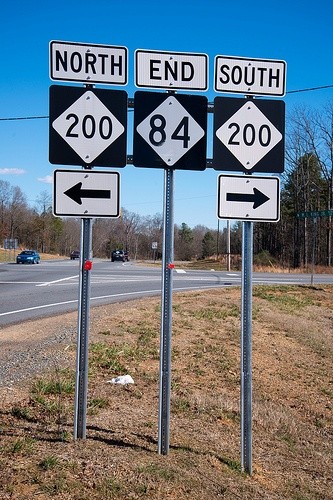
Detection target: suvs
<box><xmin>111</xmin><ymin>250</ymin><xmax>130</xmax><ymax>262</ymax></box>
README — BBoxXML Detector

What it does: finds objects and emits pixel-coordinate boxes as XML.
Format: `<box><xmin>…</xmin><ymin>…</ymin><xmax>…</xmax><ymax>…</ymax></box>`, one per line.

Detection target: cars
<box><xmin>70</xmin><ymin>251</ymin><xmax>80</xmax><ymax>260</ymax></box>
<box><xmin>16</xmin><ymin>251</ymin><xmax>41</xmax><ymax>264</ymax></box>
